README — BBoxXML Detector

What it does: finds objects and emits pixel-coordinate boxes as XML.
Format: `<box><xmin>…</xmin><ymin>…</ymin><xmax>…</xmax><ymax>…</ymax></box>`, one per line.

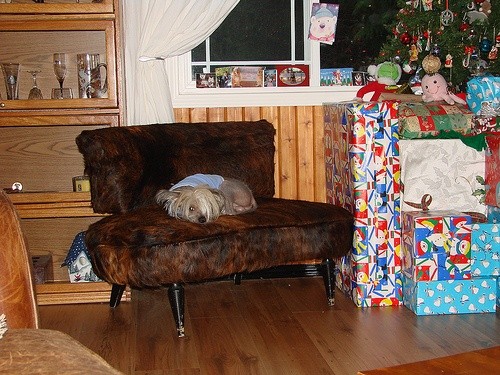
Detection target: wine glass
<box><xmin>26</xmin><ymin>69</ymin><xmax>43</xmax><ymax>99</ymax></box>
<box><xmin>52</xmin><ymin>52</ymin><xmax>68</xmax><ymax>99</ymax></box>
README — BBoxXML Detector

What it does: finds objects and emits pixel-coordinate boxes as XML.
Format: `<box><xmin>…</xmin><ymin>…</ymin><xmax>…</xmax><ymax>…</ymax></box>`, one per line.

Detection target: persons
<box><xmin>223</xmin><ymin>71</ymin><xmax>228</xmax><ymax>80</ymax></box>
<box><xmin>290</xmin><ymin>73</ymin><xmax>295</xmax><ymax>81</ymax></box>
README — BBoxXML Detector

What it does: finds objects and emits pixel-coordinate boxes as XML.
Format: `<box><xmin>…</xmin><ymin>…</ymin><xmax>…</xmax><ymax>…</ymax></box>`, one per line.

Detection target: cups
<box><xmin>76</xmin><ymin>53</ymin><xmax>107</xmax><ymax>98</ymax></box>
<box><xmin>0</xmin><ymin>63</ymin><xmax>21</xmax><ymax>100</ymax></box>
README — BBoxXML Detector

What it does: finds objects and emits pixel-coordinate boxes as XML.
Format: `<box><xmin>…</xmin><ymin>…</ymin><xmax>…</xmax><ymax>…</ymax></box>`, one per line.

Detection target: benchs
<box><xmin>75</xmin><ymin>120</ymin><xmax>355</xmax><ymax>338</ymax></box>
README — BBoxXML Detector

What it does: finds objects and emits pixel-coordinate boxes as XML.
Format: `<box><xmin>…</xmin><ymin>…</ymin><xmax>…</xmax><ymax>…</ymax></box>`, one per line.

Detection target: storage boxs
<box><xmin>322</xmin><ymin>101</ymin><xmax>500</xmax><ymax>317</ymax></box>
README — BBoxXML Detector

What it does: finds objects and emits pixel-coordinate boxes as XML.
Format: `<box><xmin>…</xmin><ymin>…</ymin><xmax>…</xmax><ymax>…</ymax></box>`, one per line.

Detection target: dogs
<box><xmin>154</xmin><ymin>171</ymin><xmax>257</xmax><ymax>226</ymax></box>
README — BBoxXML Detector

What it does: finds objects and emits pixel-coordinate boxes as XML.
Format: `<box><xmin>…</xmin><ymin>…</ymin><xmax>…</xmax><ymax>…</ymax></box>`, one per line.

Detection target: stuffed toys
<box><xmin>353</xmin><ymin>61</ymin><xmax>402</xmax><ymax>101</ymax></box>
<box><xmin>421</xmin><ymin>73</ymin><xmax>466</xmax><ymax>105</ymax></box>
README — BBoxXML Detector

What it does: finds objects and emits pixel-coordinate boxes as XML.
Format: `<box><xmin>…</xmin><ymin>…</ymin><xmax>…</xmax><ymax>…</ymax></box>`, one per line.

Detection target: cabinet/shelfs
<box><xmin>0</xmin><ymin>0</ymin><xmax>132</xmax><ymax>305</ymax></box>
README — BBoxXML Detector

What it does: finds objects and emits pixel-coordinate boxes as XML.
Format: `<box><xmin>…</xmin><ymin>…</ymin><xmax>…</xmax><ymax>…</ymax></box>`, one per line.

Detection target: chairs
<box><xmin>0</xmin><ymin>188</ymin><xmax>125</xmax><ymax>375</ymax></box>
